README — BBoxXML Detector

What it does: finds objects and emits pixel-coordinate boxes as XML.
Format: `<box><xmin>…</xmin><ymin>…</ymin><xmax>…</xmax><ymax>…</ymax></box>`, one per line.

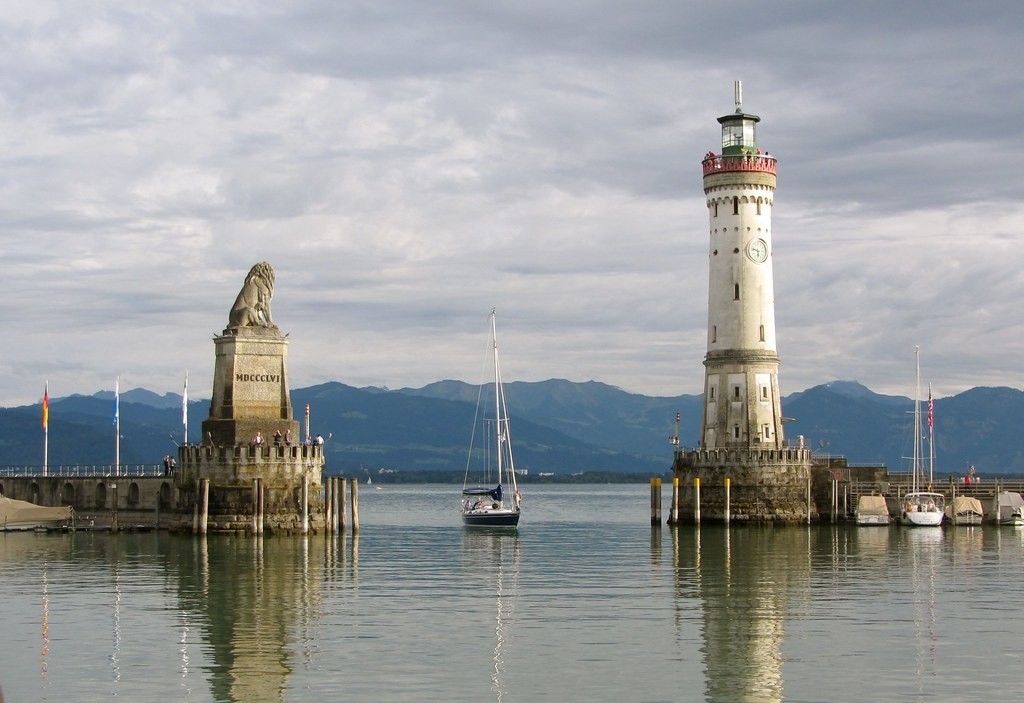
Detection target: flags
<box><xmin>112</xmin><ymin>381</ymin><xmax>117</xmax><ymax>427</ymax></box>
<box><xmin>41</xmin><ymin>384</ymin><xmax>48</xmax><ymax>429</ymax></box>
<box><xmin>928</xmin><ymin>382</ymin><xmax>933</xmax><ymax>427</ymax></box>
<box><xmin>181</xmin><ymin>373</ymin><xmax>187</xmax><ymax>424</ymax></box>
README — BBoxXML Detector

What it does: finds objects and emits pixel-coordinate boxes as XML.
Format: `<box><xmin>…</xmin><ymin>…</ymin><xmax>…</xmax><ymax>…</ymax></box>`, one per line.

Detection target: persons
<box><xmin>764</xmin><ymin>152</ymin><xmax>771</xmax><ymax>167</ymax></box>
<box><xmin>965</xmin><ymin>474</ymin><xmax>970</xmax><ymax>492</ymax></box>
<box><xmin>747</xmin><ymin>149</ymin><xmax>752</xmax><ymax>165</ymax></box>
<box><xmin>709</xmin><ymin>151</ymin><xmax>717</xmax><ymax>169</ymax></box>
<box><xmin>740</xmin><ymin>146</ymin><xmax>745</xmax><ymax>159</ymax></box>
<box><xmin>163</xmin><ymin>454</ymin><xmax>169</xmax><ymax>477</ymax></box>
<box><xmin>272</xmin><ymin>429</ymin><xmax>281</xmax><ymax>445</ymax></box>
<box><xmin>253</xmin><ymin>432</ymin><xmax>265</xmax><ymax>444</ymax></box>
<box><xmin>305</xmin><ymin>435</ymin><xmax>311</xmax><ymax>445</ymax></box>
<box><xmin>169</xmin><ymin>456</ymin><xmax>176</xmax><ymax>476</ymax></box>
<box><xmin>704</xmin><ymin>154</ymin><xmax>710</xmax><ymax>159</ymax></box>
<box><xmin>756</xmin><ymin>148</ymin><xmax>761</xmax><ymax>163</ymax></box>
<box><xmin>316</xmin><ymin>434</ymin><xmax>324</xmax><ymax>445</ymax></box>
<box><xmin>284</xmin><ymin>430</ymin><xmax>292</xmax><ymax>445</ymax></box>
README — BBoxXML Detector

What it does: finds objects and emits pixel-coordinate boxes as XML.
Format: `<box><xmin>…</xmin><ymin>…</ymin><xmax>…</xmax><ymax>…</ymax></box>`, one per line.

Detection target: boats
<box><xmin>986</xmin><ymin>490</ymin><xmax>1024</xmax><ymax>525</ymax></box>
<box><xmin>46</xmin><ymin>523</ymin><xmax>170</xmax><ymax>533</ymax></box>
<box><xmin>459</xmin><ymin>307</ymin><xmax>521</xmax><ymax>526</ymax></box>
<box><xmin>943</xmin><ymin>495</ymin><xmax>983</xmax><ymax>526</ymax></box>
<box><xmin>901</xmin><ymin>491</ymin><xmax>945</xmax><ymax>526</ymax></box>
<box><xmin>854</xmin><ymin>495</ymin><xmax>890</xmax><ymax>526</ymax></box>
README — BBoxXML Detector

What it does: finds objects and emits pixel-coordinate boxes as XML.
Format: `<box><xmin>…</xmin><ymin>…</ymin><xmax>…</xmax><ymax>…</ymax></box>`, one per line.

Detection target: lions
<box><xmin>227</xmin><ymin>261</ymin><xmax>278</xmax><ymax>329</ymax></box>
